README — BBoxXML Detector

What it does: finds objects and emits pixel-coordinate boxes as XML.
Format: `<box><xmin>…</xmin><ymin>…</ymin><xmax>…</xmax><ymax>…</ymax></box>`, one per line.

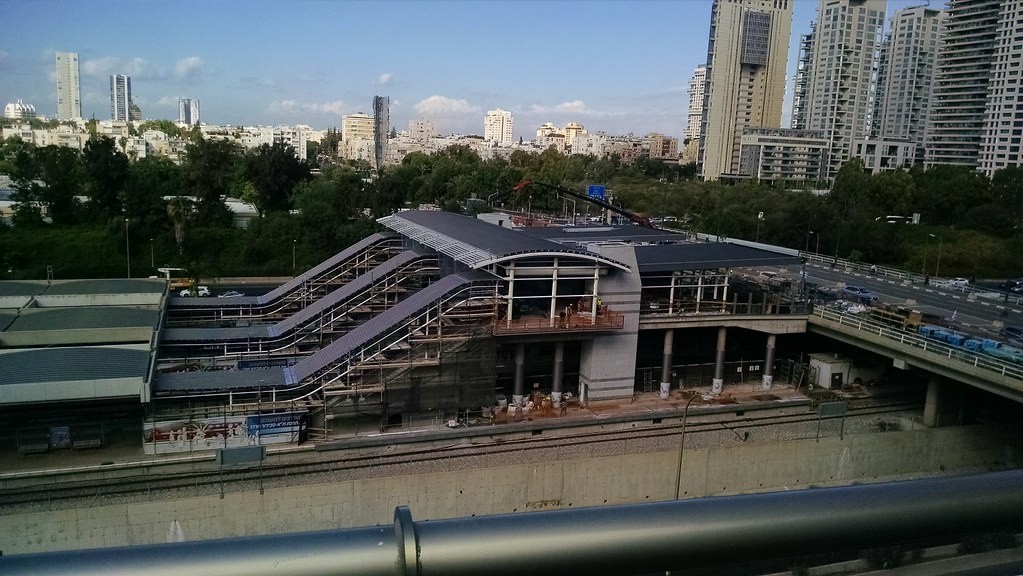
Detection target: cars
<box><xmin>218</xmin><ymin>289</ymin><xmax>246</xmax><ymax>299</ymax></box>
<box><xmin>180</xmin><ymin>286</ymin><xmax>211</xmax><ymax>297</ymax></box>
<box><xmin>479</xmin><ymin>179</ymin><xmax>1023</xmax><ymax>368</ymax></box>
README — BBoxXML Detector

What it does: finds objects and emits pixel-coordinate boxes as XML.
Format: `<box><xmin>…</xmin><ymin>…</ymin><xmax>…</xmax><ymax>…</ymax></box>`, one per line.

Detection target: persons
<box><xmin>924</xmin><ymin>272</ymin><xmax>930</xmax><ymax>286</ymax></box>
<box><xmin>567</xmin><ymin>303</ymin><xmax>573</xmax><ymax>323</ymax></box>
<box><xmin>596</xmin><ymin>298</ymin><xmax>604</xmax><ymax>315</ymax></box>
<box><xmin>520</xmin><ymin>390</ymin><xmax>573</xmax><ymax>416</ymax></box>
<box><xmin>867</xmin><ymin>264</ymin><xmax>877</xmax><ymax>277</ymax></box>
<box><xmin>557</xmin><ymin>308</ymin><xmax>566</xmax><ymax>326</ymax></box>
